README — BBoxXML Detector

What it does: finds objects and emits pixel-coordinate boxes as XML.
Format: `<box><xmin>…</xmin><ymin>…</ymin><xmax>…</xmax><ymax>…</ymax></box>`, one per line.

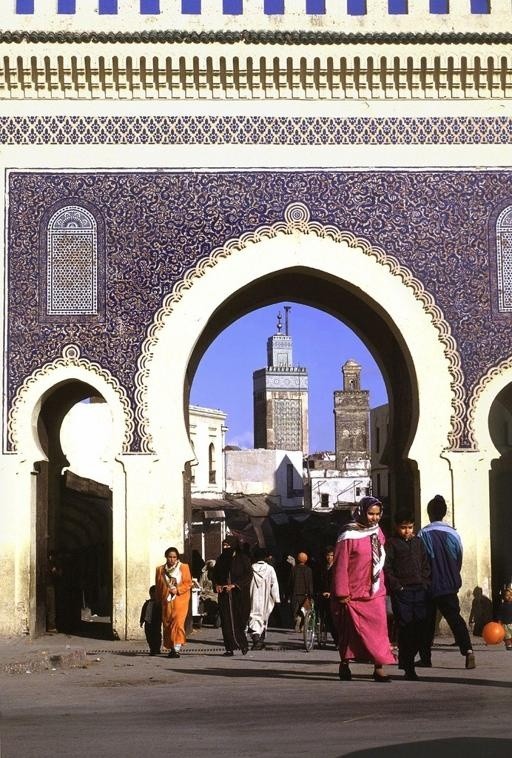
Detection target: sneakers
<box><xmin>169</xmin><ymin>652</ymin><xmax>180</xmax><ymax>658</ymax></box>
<box><xmin>339</xmin><ymin>668</ymin><xmax>351</xmax><ymax>680</ymax></box>
<box><xmin>414</xmin><ymin>659</ymin><xmax>433</xmax><ymax>668</ymax></box>
<box><xmin>223</xmin><ymin>640</ymin><xmax>265</xmax><ymax>656</ymax></box>
<box><xmin>465</xmin><ymin>652</ymin><xmax>476</xmax><ymax>669</ymax></box>
<box><xmin>374</xmin><ymin>672</ymin><xmax>390</xmax><ymax>682</ymax></box>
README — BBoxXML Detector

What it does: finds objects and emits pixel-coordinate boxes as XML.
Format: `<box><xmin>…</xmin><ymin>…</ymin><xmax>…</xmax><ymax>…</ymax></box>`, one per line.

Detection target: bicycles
<box><xmin>296</xmin><ymin>592</ymin><xmax>328</xmax><ymax>651</ymax></box>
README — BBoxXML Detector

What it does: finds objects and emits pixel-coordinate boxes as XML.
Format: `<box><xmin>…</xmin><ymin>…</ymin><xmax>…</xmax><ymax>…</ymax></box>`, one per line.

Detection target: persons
<box><xmin>191</xmin><ymin>551</ymin><xmax>313</xmax><ymax>634</ymax></box>
<box><xmin>154</xmin><ymin>546</ymin><xmax>194</xmax><ymax>659</ymax></box>
<box><xmin>208</xmin><ymin>535</ymin><xmax>251</xmax><ymax>660</ymax></box>
<box><xmin>382</xmin><ymin>507</ymin><xmax>430</xmax><ymax>682</ymax></box>
<box><xmin>415</xmin><ymin>491</ymin><xmax>477</xmax><ymax>672</ymax></box>
<box><xmin>497</xmin><ymin>587</ymin><xmax>512</xmax><ymax>653</ymax></box>
<box><xmin>311</xmin><ymin>544</ymin><xmax>340</xmax><ymax>652</ymax></box>
<box><xmin>248</xmin><ymin>550</ymin><xmax>283</xmax><ymax>652</ymax></box>
<box><xmin>138</xmin><ymin>583</ymin><xmax>163</xmax><ymax>655</ymax></box>
<box><xmin>328</xmin><ymin>492</ymin><xmax>399</xmax><ymax>682</ymax></box>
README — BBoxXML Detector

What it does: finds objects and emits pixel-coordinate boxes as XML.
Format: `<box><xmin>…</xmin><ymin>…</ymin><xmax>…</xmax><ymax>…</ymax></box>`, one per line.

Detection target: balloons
<box><xmin>482</xmin><ymin>621</ymin><xmax>505</xmax><ymax>645</ymax></box>
